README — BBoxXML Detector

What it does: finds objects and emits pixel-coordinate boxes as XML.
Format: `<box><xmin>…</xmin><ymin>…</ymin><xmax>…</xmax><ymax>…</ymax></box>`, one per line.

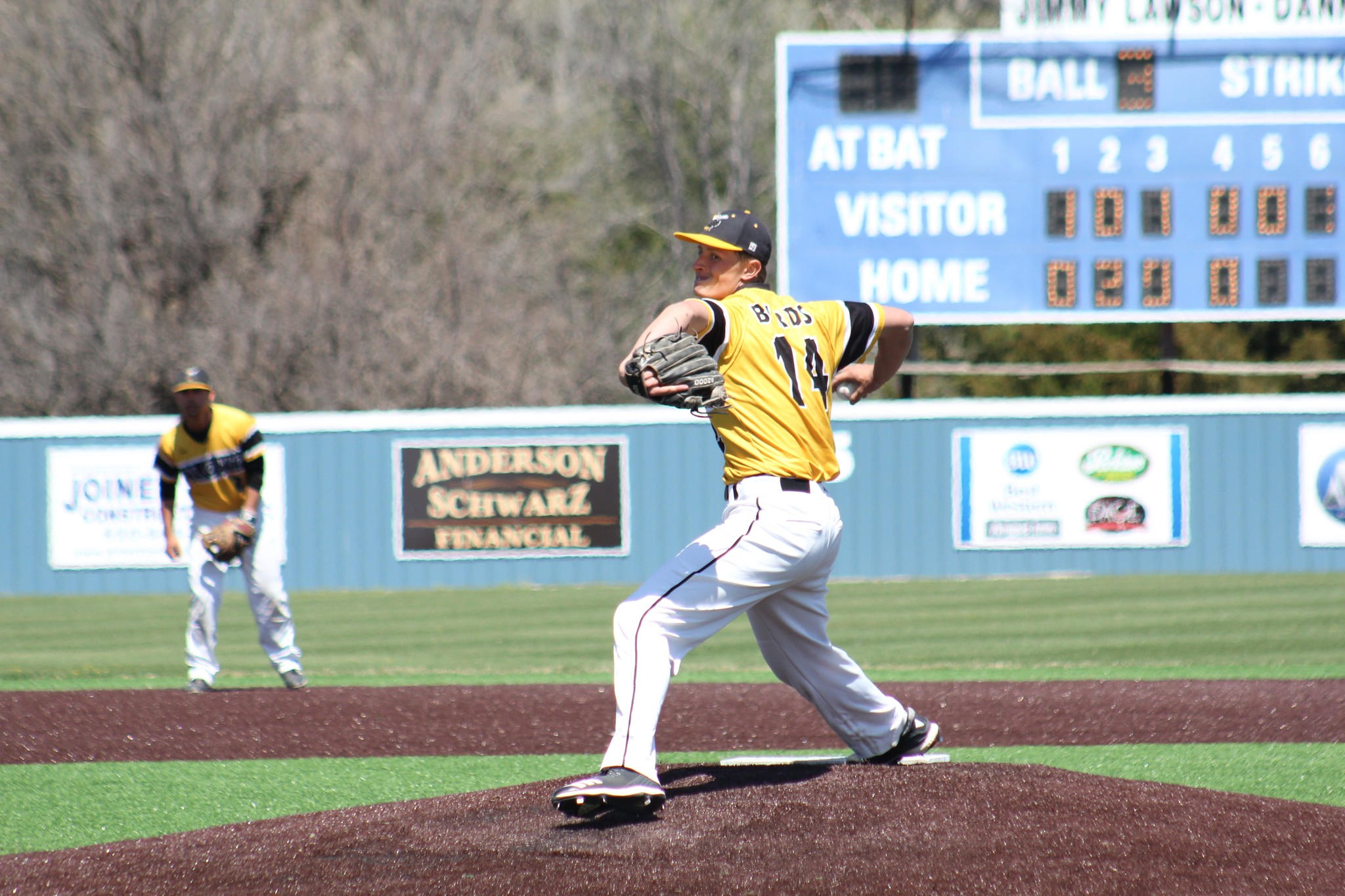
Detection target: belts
<box><xmin>724</xmin><ymin>479</ymin><xmax>811</xmax><ymax>500</ymax></box>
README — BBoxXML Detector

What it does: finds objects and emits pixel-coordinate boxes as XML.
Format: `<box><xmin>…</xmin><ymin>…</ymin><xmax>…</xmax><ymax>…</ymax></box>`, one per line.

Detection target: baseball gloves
<box><xmin>624</xmin><ymin>331</ymin><xmax>727</xmax><ymax>409</ymax></box>
<box><xmin>200</xmin><ymin>517</ymin><xmax>256</xmax><ymax>564</ymax></box>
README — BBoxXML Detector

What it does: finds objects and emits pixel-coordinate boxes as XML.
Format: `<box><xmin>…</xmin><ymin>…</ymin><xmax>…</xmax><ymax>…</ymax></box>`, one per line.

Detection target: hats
<box><xmin>172</xmin><ymin>365</ymin><xmax>211</xmax><ymax>393</ymax></box>
<box><xmin>674</xmin><ymin>210</ymin><xmax>771</xmax><ymax>267</ymax></box>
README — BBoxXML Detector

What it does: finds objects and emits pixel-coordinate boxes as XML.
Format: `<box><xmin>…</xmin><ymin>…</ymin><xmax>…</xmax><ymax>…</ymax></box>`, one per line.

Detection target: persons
<box><xmin>546</xmin><ymin>210</ymin><xmax>942</xmax><ymax>818</ymax></box>
<box><xmin>153</xmin><ymin>365</ymin><xmax>312</xmax><ymax>692</ymax></box>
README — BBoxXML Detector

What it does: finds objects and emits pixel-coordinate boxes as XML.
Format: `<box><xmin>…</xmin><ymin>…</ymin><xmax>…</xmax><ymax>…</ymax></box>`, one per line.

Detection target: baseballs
<box><xmin>836</xmin><ymin>381</ymin><xmax>860</xmax><ymax>401</ymax></box>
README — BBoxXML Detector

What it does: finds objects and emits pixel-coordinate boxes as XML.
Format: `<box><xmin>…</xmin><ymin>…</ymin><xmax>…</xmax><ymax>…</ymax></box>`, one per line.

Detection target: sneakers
<box><xmin>845</xmin><ymin>708</ymin><xmax>941</xmax><ymax>766</ymax></box>
<box><xmin>552</xmin><ymin>766</ymin><xmax>667</xmax><ymax>818</ymax></box>
<box><xmin>282</xmin><ymin>669</ymin><xmax>307</xmax><ymax>689</ymax></box>
<box><xmin>187</xmin><ymin>678</ymin><xmax>211</xmax><ymax>694</ymax></box>
